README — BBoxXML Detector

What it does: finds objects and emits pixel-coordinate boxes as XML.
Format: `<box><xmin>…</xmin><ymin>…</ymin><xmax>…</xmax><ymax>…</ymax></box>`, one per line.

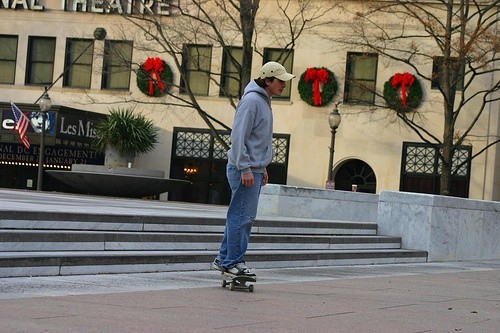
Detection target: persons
<box><xmin>211</xmin><ymin>61</ymin><xmax>296</xmax><ymax>275</ymax></box>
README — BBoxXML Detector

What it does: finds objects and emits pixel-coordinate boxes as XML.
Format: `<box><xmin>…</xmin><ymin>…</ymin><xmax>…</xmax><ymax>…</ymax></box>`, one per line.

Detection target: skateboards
<box><xmin>220</xmin><ymin>272</ymin><xmax>257</xmax><ymax>292</ymax></box>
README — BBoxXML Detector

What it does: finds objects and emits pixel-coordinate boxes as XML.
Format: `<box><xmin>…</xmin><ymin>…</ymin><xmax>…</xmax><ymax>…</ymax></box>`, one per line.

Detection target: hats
<box><xmin>259</xmin><ymin>61</ymin><xmax>296</xmax><ymax>81</ymax></box>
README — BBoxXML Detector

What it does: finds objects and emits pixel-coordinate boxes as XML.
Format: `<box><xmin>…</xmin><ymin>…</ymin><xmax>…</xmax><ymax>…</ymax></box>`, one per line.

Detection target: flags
<box><xmin>10</xmin><ymin>101</ymin><xmax>31</xmax><ymax>151</ymax></box>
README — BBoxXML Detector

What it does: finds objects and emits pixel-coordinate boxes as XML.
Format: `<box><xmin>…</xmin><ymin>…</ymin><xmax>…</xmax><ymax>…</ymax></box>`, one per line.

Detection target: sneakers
<box><xmin>227</xmin><ymin>261</ymin><xmax>250</xmax><ymax>274</ymax></box>
<box><xmin>212</xmin><ymin>257</ymin><xmax>226</xmax><ymax>273</ymax></box>
